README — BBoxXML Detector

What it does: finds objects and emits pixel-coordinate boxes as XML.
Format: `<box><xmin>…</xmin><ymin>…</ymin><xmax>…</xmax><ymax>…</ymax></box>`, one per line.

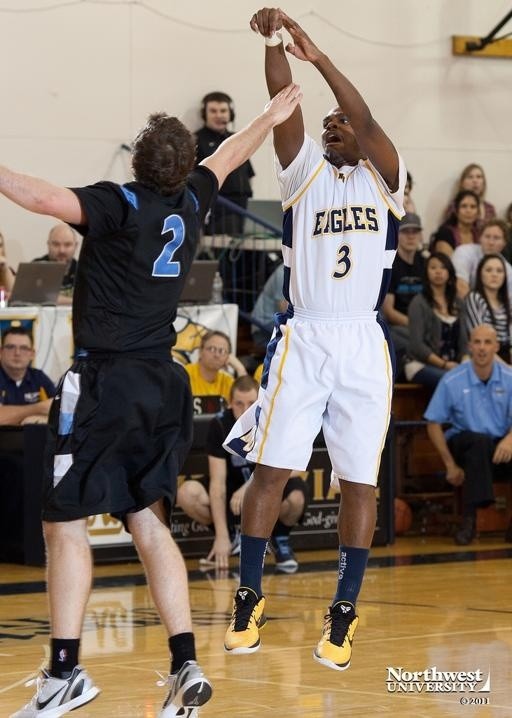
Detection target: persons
<box><xmin>221</xmin><ymin>7</ymin><xmax>408</xmax><ymax>672</ymax></box>
<box><xmin>0</xmin><ymin>81</ymin><xmax>305</xmax><ymax>718</ymax></box>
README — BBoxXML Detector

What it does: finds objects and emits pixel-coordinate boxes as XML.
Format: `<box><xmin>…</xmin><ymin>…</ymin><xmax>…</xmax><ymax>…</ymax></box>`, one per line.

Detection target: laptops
<box><xmin>5</xmin><ymin>261</ymin><xmax>67</xmax><ymax>306</ymax></box>
<box><xmin>179</xmin><ymin>261</ymin><xmax>220</xmax><ymax>302</ymax></box>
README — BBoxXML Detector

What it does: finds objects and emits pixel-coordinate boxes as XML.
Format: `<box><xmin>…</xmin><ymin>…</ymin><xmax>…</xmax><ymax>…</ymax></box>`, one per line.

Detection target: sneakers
<box><xmin>7</xmin><ymin>664</ymin><xmax>100</xmax><ymax>718</ymax></box>
<box><xmin>266</xmin><ymin>535</ymin><xmax>299</xmax><ymax>574</ymax></box>
<box><xmin>229</xmin><ymin>524</ymin><xmax>240</xmax><ymax>555</ymax></box>
<box><xmin>223</xmin><ymin>585</ymin><xmax>269</xmax><ymax>655</ymax></box>
<box><xmin>311</xmin><ymin>599</ymin><xmax>359</xmax><ymax>672</ymax></box>
<box><xmin>155</xmin><ymin>659</ymin><xmax>212</xmax><ymax>717</ymax></box>
<box><xmin>455</xmin><ymin>511</ymin><xmax>477</xmax><ymax>545</ymax></box>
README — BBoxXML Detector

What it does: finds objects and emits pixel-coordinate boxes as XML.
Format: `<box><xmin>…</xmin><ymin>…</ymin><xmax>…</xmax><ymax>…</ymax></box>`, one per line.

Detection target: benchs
<box><xmin>389</xmin><ymin>381</ymin><xmax>512</xmax><ymax>540</ymax></box>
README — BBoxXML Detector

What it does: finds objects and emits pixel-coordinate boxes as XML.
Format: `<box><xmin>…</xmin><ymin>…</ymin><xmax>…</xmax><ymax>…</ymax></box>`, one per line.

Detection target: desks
<box><xmin>1</xmin><ymin>280</ymin><xmax>242</xmax><ymax>412</ymax></box>
<box><xmin>200</xmin><ymin>235</ymin><xmax>285</xmax><ymax>254</ymax></box>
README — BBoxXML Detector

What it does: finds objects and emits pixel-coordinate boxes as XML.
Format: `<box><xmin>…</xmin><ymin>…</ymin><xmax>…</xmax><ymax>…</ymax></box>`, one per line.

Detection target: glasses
<box><xmin>205</xmin><ymin>346</ymin><xmax>229</xmax><ymax>355</ymax></box>
<box><xmin>0</xmin><ymin>343</ymin><xmax>32</xmax><ymax>351</ymax></box>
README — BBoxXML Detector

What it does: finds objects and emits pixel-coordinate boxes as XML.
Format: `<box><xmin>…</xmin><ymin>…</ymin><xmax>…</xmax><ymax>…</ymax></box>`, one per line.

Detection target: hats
<box><xmin>399</xmin><ymin>213</ymin><xmax>422</xmax><ymax>230</ymax></box>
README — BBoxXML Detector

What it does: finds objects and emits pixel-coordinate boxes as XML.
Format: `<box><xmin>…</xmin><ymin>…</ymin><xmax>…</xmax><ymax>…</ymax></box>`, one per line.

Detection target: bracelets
<box><xmin>263</xmin><ymin>28</ymin><xmax>284</xmax><ymax>46</ymax></box>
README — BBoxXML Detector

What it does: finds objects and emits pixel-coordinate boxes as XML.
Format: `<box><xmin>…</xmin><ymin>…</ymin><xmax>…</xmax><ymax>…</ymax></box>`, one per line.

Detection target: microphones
<box><xmin>222</xmin><ymin>120</ymin><xmax>235</xmax><ymax>126</ymax></box>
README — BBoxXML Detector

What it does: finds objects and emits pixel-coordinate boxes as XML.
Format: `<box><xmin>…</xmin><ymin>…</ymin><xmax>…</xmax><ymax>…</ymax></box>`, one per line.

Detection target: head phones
<box><xmin>199</xmin><ymin>92</ymin><xmax>237</xmax><ymax>117</ymax></box>
